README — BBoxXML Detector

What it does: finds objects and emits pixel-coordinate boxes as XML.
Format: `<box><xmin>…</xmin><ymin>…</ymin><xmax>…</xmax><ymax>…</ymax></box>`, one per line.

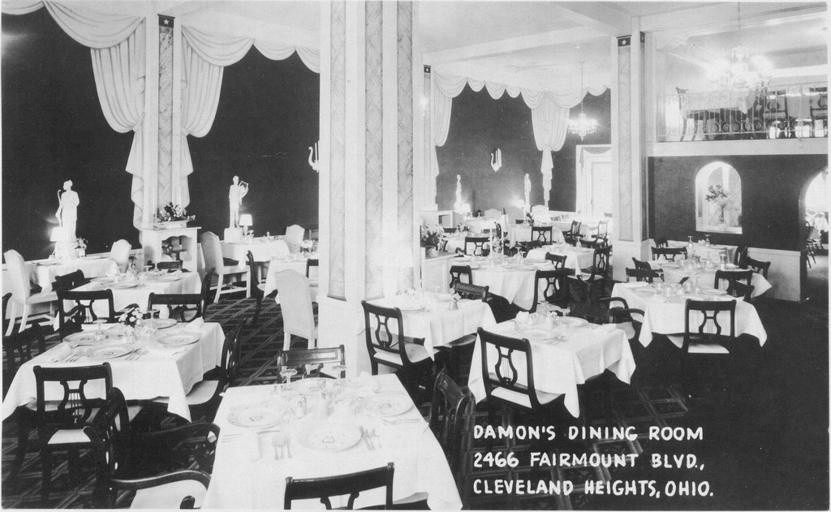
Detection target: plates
<box><xmin>88</xmin><ymin>276</ymin><xmax>110</xmax><ymax>284</ymax></box>
<box><xmin>113</xmin><ymin>282</ymin><xmax>136</xmax><ymax>289</ymax></box>
<box><xmin>86</xmin><ymin>343</ymin><xmax>134</xmax><ymax>360</ymax></box>
<box><xmin>355</xmin><ymin>389</ymin><xmax>415</xmax><ymax>419</ymax></box>
<box><xmin>161</xmin><ymin>271</ymin><xmax>181</xmax><ymax>282</ymax></box>
<box><xmin>302</xmin><ymin>420</ymin><xmax>365</xmax><ymax>450</ymax></box>
<box><xmin>157</xmin><ymin>316</ymin><xmax>177</xmax><ymax>328</ymax></box>
<box><xmin>61</xmin><ymin>332</ymin><xmax>97</xmax><ymax>346</ymax></box>
<box><xmin>156</xmin><ymin>332</ymin><xmax>201</xmax><ymax>346</ymax></box>
<box><xmin>228</xmin><ymin>400</ymin><xmax>286</xmax><ymax>430</ymax></box>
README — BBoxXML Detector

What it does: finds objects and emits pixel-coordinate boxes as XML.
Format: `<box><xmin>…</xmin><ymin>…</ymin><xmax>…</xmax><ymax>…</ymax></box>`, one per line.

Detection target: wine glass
<box><xmin>281</xmin><ymin>360</ymin><xmax>349</xmax><ymax>418</ymax></box>
<box><xmin>519</xmin><ymin>306</ymin><xmax>566</xmax><ymax>345</ymax></box>
<box><xmin>650</xmin><ymin>271</ymin><xmax>705</xmax><ymax>302</ymax></box>
<box><xmin>95</xmin><ymin>310</ymin><xmax>157</xmax><ymax>346</ymax></box>
<box><xmin>244</xmin><ymin>229</ymin><xmax>271</xmax><ymax>243</ymax></box>
<box><xmin>574</xmin><ymin>234</ymin><xmax>584</xmax><ymax>250</ymax></box>
<box><xmin>677</xmin><ymin>232</ymin><xmax>728</xmax><ymax>271</ymax></box>
<box><xmin>488</xmin><ymin>247</ymin><xmax>526</xmax><ymax>272</ymax></box>
<box><xmin>288</xmin><ymin>240</ymin><xmax>317</xmax><ymax>261</ymax></box>
<box><xmin>110</xmin><ymin>258</ymin><xmax>159</xmax><ymax>286</ymax></box>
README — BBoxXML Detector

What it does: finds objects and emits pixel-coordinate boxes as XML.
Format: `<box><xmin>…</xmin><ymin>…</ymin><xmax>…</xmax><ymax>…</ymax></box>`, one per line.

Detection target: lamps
<box><xmin>701</xmin><ymin>2</ymin><xmax>787</xmax><ymax>101</ymax></box>
<box><xmin>565</xmin><ymin>57</ymin><xmax>602</xmax><ymax>142</ymax></box>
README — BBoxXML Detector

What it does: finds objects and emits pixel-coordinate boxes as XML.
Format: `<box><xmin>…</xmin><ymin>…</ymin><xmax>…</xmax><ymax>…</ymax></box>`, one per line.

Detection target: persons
<box><xmin>54</xmin><ymin>179</ymin><xmax>80</xmax><ymax>230</ymax></box>
<box><xmin>523</xmin><ymin>172</ymin><xmax>532</xmax><ymax>203</ymax></box>
<box><xmin>455</xmin><ymin>174</ymin><xmax>463</xmax><ymax>200</ymax></box>
<box><xmin>228</xmin><ymin>175</ymin><xmax>250</xmax><ymax>227</ymax></box>
